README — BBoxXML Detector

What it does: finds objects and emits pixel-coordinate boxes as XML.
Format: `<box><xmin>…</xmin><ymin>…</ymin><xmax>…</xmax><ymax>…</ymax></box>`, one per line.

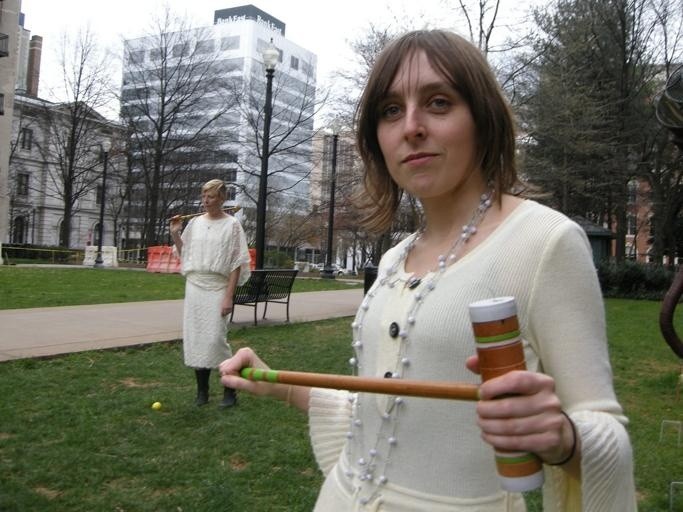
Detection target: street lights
<box><xmin>94</xmin><ymin>135</ymin><xmax>112</xmax><ymax>267</ymax></box>
<box><xmin>254</xmin><ymin>37</ymin><xmax>280</xmax><ymax>271</ymax></box>
<box><xmin>320</xmin><ymin>114</ymin><xmax>344</xmax><ymax>280</ymax></box>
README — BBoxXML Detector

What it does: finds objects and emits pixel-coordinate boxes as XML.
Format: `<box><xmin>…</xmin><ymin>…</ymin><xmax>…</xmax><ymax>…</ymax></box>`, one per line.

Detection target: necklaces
<box><xmin>343</xmin><ymin>185</ymin><xmax>500</xmax><ymax>510</ymax></box>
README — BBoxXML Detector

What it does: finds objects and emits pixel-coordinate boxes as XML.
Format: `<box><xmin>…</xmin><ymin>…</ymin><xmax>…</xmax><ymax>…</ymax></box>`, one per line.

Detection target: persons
<box><xmin>216</xmin><ymin>28</ymin><xmax>640</xmax><ymax>512</ymax></box>
<box><xmin>168</xmin><ymin>179</ymin><xmax>251</xmax><ymax>410</ymax></box>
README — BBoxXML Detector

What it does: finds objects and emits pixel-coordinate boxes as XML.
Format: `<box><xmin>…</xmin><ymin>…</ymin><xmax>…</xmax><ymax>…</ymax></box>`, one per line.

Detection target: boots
<box><xmin>219</xmin><ymin>386</ymin><xmax>237</xmax><ymax>408</ymax></box>
<box><xmin>193</xmin><ymin>368</ymin><xmax>211</xmax><ymax>407</ymax></box>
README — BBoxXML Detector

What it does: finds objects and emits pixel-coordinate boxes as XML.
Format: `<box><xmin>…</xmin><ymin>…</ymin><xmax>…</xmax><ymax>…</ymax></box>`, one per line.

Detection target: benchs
<box><xmin>230</xmin><ymin>270</ymin><xmax>299</xmax><ymax>325</ymax></box>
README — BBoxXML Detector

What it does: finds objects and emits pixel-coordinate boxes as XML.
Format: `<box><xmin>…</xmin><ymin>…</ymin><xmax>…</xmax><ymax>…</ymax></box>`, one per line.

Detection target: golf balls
<box><xmin>152</xmin><ymin>402</ymin><xmax>162</xmax><ymax>410</ymax></box>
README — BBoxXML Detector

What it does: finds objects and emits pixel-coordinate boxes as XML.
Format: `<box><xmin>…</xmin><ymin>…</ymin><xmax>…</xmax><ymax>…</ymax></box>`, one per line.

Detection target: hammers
<box><xmin>239</xmin><ymin>296</ymin><xmax>547</xmax><ymax>492</ymax></box>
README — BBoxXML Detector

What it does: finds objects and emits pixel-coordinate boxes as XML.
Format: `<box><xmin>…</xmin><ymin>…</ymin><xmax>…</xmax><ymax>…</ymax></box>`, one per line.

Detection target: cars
<box><xmin>318</xmin><ymin>262</ymin><xmax>352</xmax><ymax>277</ymax></box>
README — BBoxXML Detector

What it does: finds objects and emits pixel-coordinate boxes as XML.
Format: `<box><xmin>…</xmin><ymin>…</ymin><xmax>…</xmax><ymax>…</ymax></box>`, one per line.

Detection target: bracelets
<box><xmin>284</xmin><ymin>383</ymin><xmax>293</xmax><ymax>410</ymax></box>
<box><xmin>540</xmin><ymin>408</ymin><xmax>577</xmax><ymax>467</ymax></box>
